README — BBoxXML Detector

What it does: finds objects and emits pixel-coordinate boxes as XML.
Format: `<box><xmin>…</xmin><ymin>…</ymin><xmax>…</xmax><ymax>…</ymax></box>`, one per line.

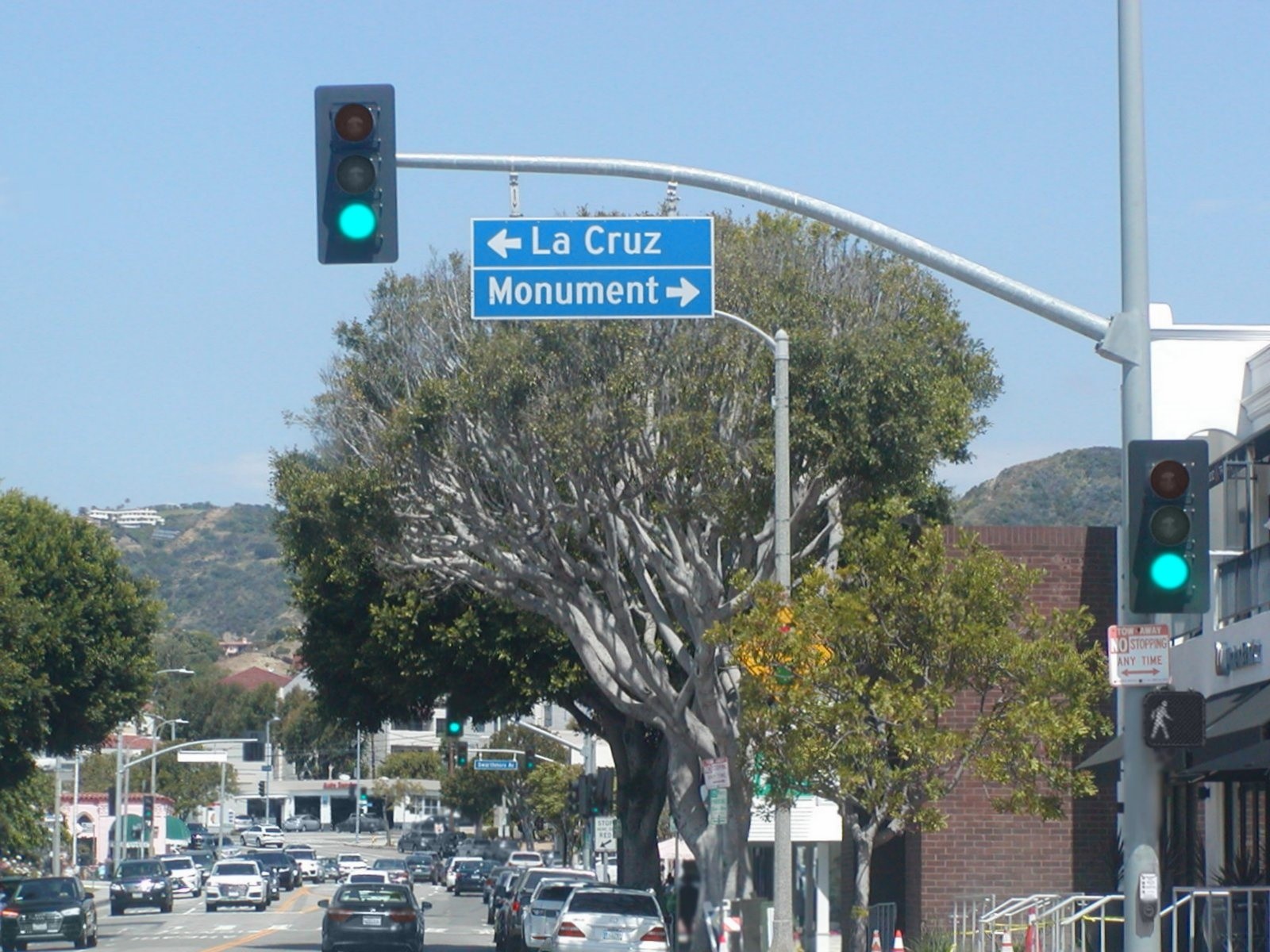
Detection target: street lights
<box><xmin>265</xmin><ymin>717</ymin><xmax>281</xmax><ymax>824</ymax></box>
<box><xmin>121</xmin><ymin>736</ymin><xmax>161</xmax><ymax>862</ymax></box>
<box><xmin>112</xmin><ymin>668</ymin><xmax>195</xmax><ymax>881</ymax></box>
<box><xmin>148</xmin><ymin>719</ymin><xmax>190</xmax><ymax>859</ymax></box>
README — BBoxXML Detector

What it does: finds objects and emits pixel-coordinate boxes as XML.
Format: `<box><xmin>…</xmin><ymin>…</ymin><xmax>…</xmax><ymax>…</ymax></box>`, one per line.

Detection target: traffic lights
<box><xmin>346</xmin><ymin>784</ymin><xmax>355</xmax><ymax>801</ymax></box>
<box><xmin>313</xmin><ymin>83</ymin><xmax>399</xmax><ymax>266</ymax></box>
<box><xmin>447</xmin><ymin>707</ymin><xmax>465</xmax><ymax>739</ymax></box>
<box><xmin>524</xmin><ymin>744</ymin><xmax>536</xmax><ymax>772</ymax></box>
<box><xmin>361</xmin><ymin>787</ymin><xmax>368</xmax><ymax>801</ymax></box>
<box><xmin>1124</xmin><ymin>438</ymin><xmax>1219</xmax><ymax>636</ymax></box>
<box><xmin>1143</xmin><ymin>687</ymin><xmax>1211</xmax><ymax>751</ymax></box>
<box><xmin>258</xmin><ymin>780</ymin><xmax>267</xmax><ymax>796</ymax></box>
<box><xmin>458</xmin><ymin>742</ymin><xmax>468</xmax><ymax>767</ymax></box>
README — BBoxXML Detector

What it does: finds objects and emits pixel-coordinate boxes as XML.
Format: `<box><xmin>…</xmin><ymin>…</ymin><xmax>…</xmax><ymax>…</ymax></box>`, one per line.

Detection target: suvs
<box><xmin>109</xmin><ymin>860</ymin><xmax>174</xmax><ymax>916</ymax></box>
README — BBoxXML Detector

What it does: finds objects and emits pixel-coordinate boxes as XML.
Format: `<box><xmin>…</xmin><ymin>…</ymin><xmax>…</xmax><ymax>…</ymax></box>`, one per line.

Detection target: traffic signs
<box><xmin>471</xmin><ymin>216</ymin><xmax>718</xmax><ymax>322</ymax></box>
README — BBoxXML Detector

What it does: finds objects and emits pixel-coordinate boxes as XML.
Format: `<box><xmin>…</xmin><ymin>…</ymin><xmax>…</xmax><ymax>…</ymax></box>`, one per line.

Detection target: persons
<box><xmin>661</xmin><ymin>873</ymin><xmax>698</xmax><ymax>949</ymax></box>
<box><xmin>49</xmin><ymin>849</ymin><xmax>112</xmax><ymax>881</ymax></box>
<box><xmin>798</xmin><ymin>871</ymin><xmax>816</xmax><ymax>930</ymax></box>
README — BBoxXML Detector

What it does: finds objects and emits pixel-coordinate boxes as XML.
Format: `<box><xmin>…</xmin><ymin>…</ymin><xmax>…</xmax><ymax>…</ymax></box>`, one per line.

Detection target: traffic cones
<box><xmin>1000</xmin><ymin>926</ymin><xmax>1014</xmax><ymax>952</ymax></box>
<box><xmin>1025</xmin><ymin>906</ymin><xmax>1043</xmax><ymax>951</ymax></box>
<box><xmin>891</xmin><ymin>930</ymin><xmax>905</xmax><ymax>952</ymax></box>
<box><xmin>870</xmin><ymin>930</ymin><xmax>883</xmax><ymax>952</ymax></box>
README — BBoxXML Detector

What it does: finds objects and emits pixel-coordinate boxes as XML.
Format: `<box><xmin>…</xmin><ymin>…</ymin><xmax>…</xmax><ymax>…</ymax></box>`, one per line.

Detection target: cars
<box><xmin>0</xmin><ymin>876</ymin><xmax>26</xmax><ymax>912</ymax></box>
<box><xmin>537</xmin><ymin>883</ymin><xmax>669</xmax><ymax>952</ymax></box>
<box><xmin>0</xmin><ymin>876</ymin><xmax>98</xmax><ymax>951</ymax></box>
<box><xmin>522</xmin><ymin>876</ymin><xmax>612</xmax><ymax>952</ymax></box>
<box><xmin>317</xmin><ymin>882</ymin><xmax>433</xmax><ymax>952</ymax></box>
<box><xmin>160</xmin><ymin>856</ymin><xmax>206</xmax><ymax>897</ymax></box>
<box><xmin>152</xmin><ymin>813</ymin><xmax>598</xmax><ymax>952</ymax></box>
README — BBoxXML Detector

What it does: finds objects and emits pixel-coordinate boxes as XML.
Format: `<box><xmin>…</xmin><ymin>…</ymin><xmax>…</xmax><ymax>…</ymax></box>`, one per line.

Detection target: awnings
<box><xmin>165</xmin><ymin>816</ymin><xmax>191</xmax><ymax>846</ymax></box>
<box><xmin>1076</xmin><ymin>679</ymin><xmax>1270</xmax><ymax>783</ymax></box>
<box><xmin>110</xmin><ymin>814</ymin><xmax>151</xmax><ymax>848</ymax></box>
